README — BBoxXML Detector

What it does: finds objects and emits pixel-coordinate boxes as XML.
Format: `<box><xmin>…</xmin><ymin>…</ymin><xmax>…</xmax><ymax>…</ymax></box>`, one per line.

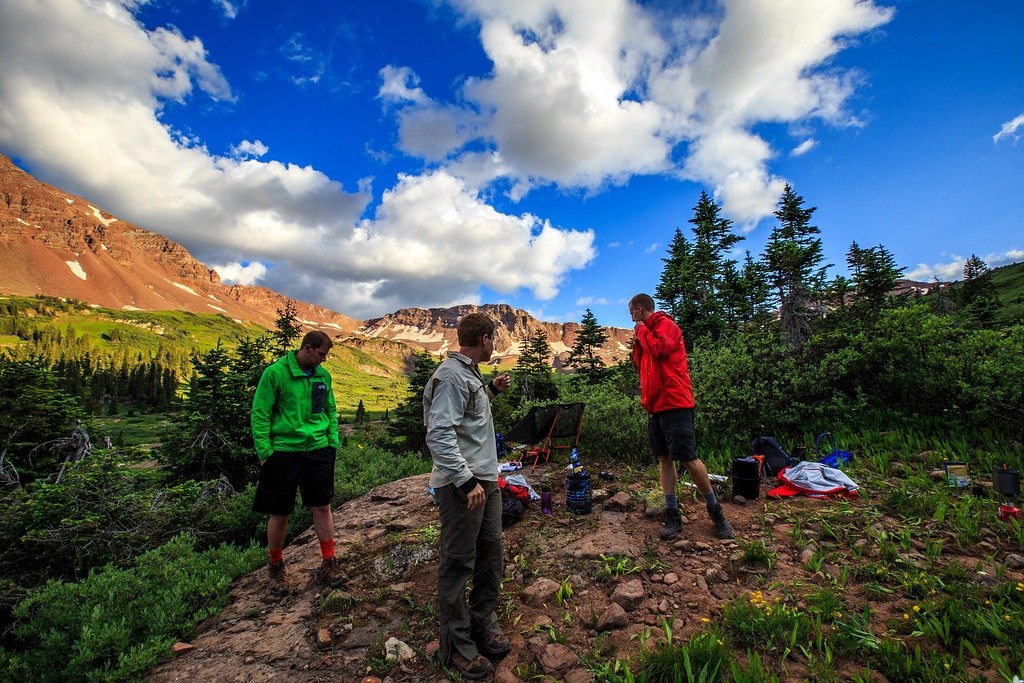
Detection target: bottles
<box><xmin>570</xmin><ymin>448</ymin><xmax>582</xmax><ymax>474</ymax></box>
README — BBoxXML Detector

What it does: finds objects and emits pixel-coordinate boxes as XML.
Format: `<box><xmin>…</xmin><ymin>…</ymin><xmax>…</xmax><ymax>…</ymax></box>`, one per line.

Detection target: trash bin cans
<box><xmin>731</xmin><ymin>455</ymin><xmax>760</xmax><ymax>499</ymax></box>
<box><xmin>564</xmin><ymin>472</ymin><xmax>592</xmax><ymax>515</ymax></box>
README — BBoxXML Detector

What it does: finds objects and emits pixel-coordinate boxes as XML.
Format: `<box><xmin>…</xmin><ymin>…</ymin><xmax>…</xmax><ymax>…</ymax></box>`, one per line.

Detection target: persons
<box><xmin>249</xmin><ymin>331</ymin><xmax>348</xmax><ymax>595</ymax></box>
<box><xmin>629</xmin><ymin>293</ymin><xmax>734</xmax><ymax>540</ymax></box>
<box><xmin>420</xmin><ymin>313</ymin><xmax>512</xmax><ymax>679</ymax></box>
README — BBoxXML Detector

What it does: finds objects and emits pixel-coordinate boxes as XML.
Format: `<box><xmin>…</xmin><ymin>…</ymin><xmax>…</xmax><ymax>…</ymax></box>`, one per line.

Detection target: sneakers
<box><xmin>660</xmin><ymin>508</ymin><xmax>684</xmax><ymax>540</ymax></box>
<box><xmin>479</xmin><ymin>635</ymin><xmax>512</xmax><ymax>654</ymax></box>
<box><xmin>268</xmin><ymin>560</ymin><xmax>290</xmax><ymax>595</ymax></box>
<box><xmin>706</xmin><ymin>502</ymin><xmax>734</xmax><ymax>538</ymax></box>
<box><xmin>450</xmin><ymin>649</ymin><xmax>493</xmax><ymax>680</ymax></box>
<box><xmin>319</xmin><ymin>556</ymin><xmax>347</xmax><ymax>582</ymax></box>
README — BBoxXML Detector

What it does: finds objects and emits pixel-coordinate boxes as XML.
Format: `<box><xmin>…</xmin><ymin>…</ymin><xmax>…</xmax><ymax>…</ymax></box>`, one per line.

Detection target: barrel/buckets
<box><xmin>944</xmin><ymin>461</ymin><xmax>971</xmax><ymax>490</ymax></box>
<box><xmin>565</xmin><ymin>474</ymin><xmax>592</xmax><ymax>515</ymax></box>
<box><xmin>992</xmin><ymin>469</ymin><xmax>1020</xmax><ymax>494</ymax></box>
<box><xmin>731</xmin><ymin>455</ymin><xmax>760</xmax><ymax>500</ymax></box>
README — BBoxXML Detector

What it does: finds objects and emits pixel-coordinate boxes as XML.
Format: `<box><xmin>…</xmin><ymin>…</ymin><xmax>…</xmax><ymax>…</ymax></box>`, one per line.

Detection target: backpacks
<box><xmin>502</xmin><ymin>497</ymin><xmax>527</xmax><ymax>526</ymax></box>
<box><xmin>748</xmin><ymin>437</ymin><xmax>800</xmax><ymax>479</ymax></box>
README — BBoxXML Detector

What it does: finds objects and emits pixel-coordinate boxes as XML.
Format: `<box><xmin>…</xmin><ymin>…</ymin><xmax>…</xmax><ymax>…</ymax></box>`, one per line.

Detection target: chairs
<box><xmin>496</xmin><ymin>401</ymin><xmax>586</xmax><ymax>475</ymax></box>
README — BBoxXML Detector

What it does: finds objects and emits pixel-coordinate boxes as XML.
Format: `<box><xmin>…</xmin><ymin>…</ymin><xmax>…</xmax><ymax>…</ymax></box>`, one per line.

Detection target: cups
<box><xmin>540</xmin><ymin>487</ymin><xmax>552</xmax><ymax>514</ymax></box>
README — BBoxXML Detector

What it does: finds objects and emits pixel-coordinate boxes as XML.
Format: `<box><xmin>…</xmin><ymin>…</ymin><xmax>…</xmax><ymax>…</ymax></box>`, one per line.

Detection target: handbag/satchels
<box><xmin>566</xmin><ymin>472</ymin><xmax>592</xmax><ymax>516</ymax></box>
<box><xmin>732</xmin><ymin>456</ymin><xmax>760</xmax><ymax>499</ymax></box>
<box><xmin>817</xmin><ymin>432</ymin><xmax>853</xmax><ymax>469</ymax></box>
